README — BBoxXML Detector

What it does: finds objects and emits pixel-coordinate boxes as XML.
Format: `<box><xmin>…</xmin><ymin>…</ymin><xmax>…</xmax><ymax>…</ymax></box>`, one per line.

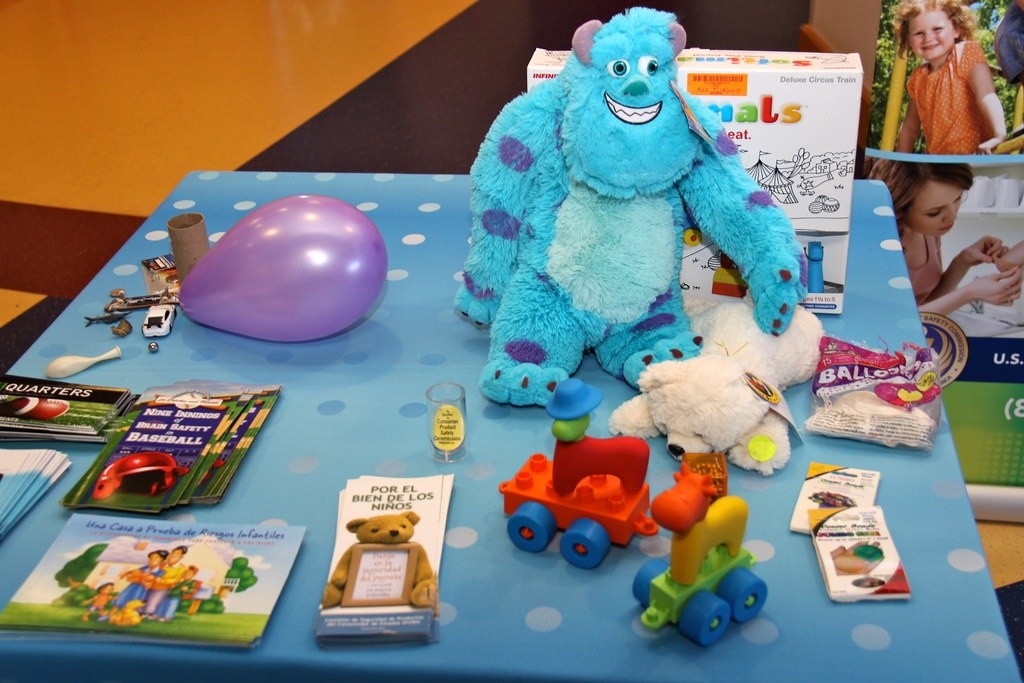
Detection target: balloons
<box><xmin>45</xmin><ymin>345</ymin><xmax>122</xmax><ymax>378</ymax></box>
<box><xmin>179</xmin><ymin>196</ymin><xmax>388</xmax><ymax>342</ymax></box>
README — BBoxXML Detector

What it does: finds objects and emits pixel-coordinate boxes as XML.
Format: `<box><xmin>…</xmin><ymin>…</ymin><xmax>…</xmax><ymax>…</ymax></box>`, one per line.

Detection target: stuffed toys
<box><xmin>450</xmin><ymin>8</ymin><xmax>803</xmax><ymax>406</ymax></box>
<box><xmin>322</xmin><ymin>510</ymin><xmax>434</xmax><ymax>608</ymax></box>
<box><xmin>607</xmin><ymin>294</ymin><xmax>824</xmax><ymax>477</ymax></box>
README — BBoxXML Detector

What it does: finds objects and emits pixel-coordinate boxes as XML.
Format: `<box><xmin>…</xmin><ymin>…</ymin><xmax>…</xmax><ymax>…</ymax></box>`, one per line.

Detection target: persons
<box><xmin>869</xmin><ymin>158</ymin><xmax>1024</xmax><ymax>316</ymax></box>
<box><xmin>893</xmin><ymin>0</ymin><xmax>1007</xmax><ymax>155</ymax></box>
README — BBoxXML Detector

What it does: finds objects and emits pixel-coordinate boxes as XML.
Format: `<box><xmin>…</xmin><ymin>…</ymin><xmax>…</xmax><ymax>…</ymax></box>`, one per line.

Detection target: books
<box><xmin>0</xmin><ymin>371</ymin><xmax>307</xmax><ymax>650</ymax></box>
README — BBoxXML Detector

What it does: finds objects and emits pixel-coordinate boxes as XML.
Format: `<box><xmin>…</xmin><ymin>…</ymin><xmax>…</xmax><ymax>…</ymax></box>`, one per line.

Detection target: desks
<box><xmin>0</xmin><ymin>172</ymin><xmax>1023</xmax><ymax>683</ymax></box>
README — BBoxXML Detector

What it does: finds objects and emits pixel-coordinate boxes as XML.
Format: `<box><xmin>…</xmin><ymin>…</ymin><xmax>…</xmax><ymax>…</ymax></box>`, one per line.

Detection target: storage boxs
<box><xmin>526</xmin><ymin>49</ymin><xmax>866</xmax><ymax>315</ymax></box>
